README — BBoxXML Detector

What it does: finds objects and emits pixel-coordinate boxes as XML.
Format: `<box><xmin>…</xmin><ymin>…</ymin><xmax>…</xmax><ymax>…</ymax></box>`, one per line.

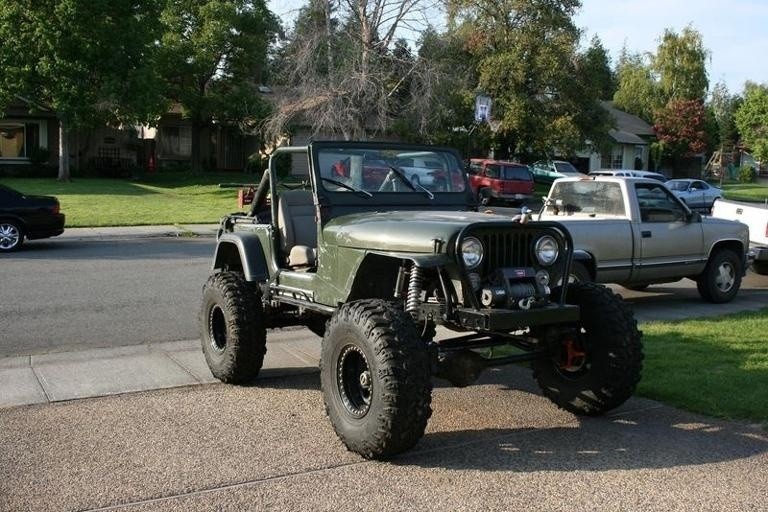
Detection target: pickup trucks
<box><xmin>506</xmin><ymin>176</ymin><xmax>753</xmax><ymax>304</ymax></box>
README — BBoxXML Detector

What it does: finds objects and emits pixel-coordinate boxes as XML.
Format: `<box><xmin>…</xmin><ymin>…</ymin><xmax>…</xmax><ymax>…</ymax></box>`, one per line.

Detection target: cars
<box><xmin>1</xmin><ymin>181</ymin><xmax>65</xmax><ymax>255</ymax></box>
<box><xmin>661</xmin><ymin>178</ymin><xmax>768</xmax><ymax>279</ymax></box>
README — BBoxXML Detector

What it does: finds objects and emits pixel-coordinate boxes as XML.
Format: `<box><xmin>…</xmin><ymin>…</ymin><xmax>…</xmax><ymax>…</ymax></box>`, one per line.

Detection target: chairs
<box><xmin>276</xmin><ymin>189</ymin><xmax>320</xmax><ymax>270</ymax></box>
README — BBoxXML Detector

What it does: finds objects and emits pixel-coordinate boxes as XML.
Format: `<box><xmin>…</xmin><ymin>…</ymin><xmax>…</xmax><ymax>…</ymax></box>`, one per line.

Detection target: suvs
<box><xmin>194</xmin><ymin>134</ymin><xmax>648</xmax><ymax>462</ymax></box>
